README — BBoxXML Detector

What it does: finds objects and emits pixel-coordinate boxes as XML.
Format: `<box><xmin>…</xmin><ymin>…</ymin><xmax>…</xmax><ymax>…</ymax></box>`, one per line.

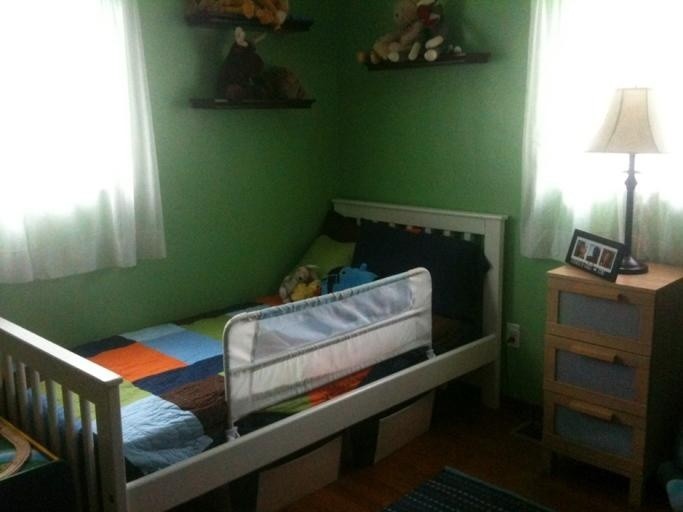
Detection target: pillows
<box><xmin>293</xmin><ymin>209</ymin><xmax>379</xmax><ymax>279</ymax></box>
<box><xmin>352</xmin><ymin>217</ymin><xmax>483</xmax><ymax>328</ymax></box>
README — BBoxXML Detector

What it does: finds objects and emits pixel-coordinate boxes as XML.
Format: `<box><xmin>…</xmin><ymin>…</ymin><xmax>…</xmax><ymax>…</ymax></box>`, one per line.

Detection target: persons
<box><xmin>599</xmin><ymin>251</ymin><xmax>615</xmax><ymax>268</ymax></box>
<box><xmin>574</xmin><ymin>241</ymin><xmax>586</xmax><ymax>259</ymax></box>
<box><xmin>587</xmin><ymin>246</ymin><xmax>601</xmax><ymax>264</ymax></box>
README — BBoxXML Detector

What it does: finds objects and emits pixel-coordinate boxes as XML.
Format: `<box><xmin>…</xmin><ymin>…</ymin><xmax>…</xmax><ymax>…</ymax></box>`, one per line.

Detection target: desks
<box><xmin>0</xmin><ymin>415</ymin><xmax>77</xmax><ymax>511</ymax></box>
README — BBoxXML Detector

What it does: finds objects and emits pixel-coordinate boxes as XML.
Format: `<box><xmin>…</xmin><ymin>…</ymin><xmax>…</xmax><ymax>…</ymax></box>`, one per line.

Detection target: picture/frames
<box><xmin>566</xmin><ymin>229</ymin><xmax>625</xmax><ymax>283</ymax></box>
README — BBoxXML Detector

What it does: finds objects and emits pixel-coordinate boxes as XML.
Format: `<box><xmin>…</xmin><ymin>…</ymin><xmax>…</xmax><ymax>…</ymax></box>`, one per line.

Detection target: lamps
<box><xmin>585</xmin><ymin>87</ymin><xmax>661</xmax><ymax>274</ymax></box>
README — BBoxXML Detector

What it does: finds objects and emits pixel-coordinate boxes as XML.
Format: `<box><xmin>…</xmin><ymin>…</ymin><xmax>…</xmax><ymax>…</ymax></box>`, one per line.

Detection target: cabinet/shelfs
<box><xmin>539</xmin><ymin>257</ymin><xmax>683</xmax><ymax>509</ymax></box>
<box><xmin>185</xmin><ymin>10</ymin><xmax>490</xmax><ymax>111</ymax></box>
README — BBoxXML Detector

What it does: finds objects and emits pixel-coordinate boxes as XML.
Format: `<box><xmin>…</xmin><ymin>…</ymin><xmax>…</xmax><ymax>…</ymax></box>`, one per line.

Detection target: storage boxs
<box><xmin>230</xmin><ymin>433</ymin><xmax>343</xmax><ymax>512</ymax></box>
<box><xmin>344</xmin><ymin>387</ymin><xmax>436</xmax><ymax>472</ymax></box>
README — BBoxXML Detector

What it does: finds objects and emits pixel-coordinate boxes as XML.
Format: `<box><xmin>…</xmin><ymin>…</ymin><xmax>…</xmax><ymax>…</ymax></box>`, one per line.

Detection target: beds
<box><xmin>0</xmin><ymin>197</ymin><xmax>509</xmax><ymax>511</ymax></box>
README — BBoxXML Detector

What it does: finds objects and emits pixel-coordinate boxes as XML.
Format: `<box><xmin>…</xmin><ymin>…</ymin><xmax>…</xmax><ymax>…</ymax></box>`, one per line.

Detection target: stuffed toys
<box><xmin>275</xmin><ymin>262</ymin><xmax>384</xmax><ymax>309</ymax></box>
<box><xmin>387</xmin><ymin>1</ymin><xmax>448</xmax><ymax>63</ymax></box>
<box><xmin>267</xmin><ymin>61</ymin><xmax>306</xmax><ymax>101</ymax></box>
<box><xmin>215</xmin><ymin>27</ymin><xmax>278</xmax><ymax>102</ymax></box>
<box><xmin>241</xmin><ymin>1</ymin><xmax>290</xmax><ymax>31</ymax></box>
<box><xmin>355</xmin><ymin>0</ymin><xmax>424</xmax><ymax>65</ymax></box>
<box><xmin>187</xmin><ymin>1</ymin><xmax>255</xmax><ymax>21</ymax></box>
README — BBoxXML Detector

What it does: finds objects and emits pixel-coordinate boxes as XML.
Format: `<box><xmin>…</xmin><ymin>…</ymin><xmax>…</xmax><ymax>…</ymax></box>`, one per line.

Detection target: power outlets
<box><xmin>504</xmin><ymin>323</ymin><xmax>520</xmax><ymax>350</ymax></box>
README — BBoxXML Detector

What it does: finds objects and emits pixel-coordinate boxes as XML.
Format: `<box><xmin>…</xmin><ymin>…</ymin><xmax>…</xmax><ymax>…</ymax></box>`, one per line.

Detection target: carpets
<box><xmin>380</xmin><ymin>464</ymin><xmax>559</xmax><ymax>511</ymax></box>
